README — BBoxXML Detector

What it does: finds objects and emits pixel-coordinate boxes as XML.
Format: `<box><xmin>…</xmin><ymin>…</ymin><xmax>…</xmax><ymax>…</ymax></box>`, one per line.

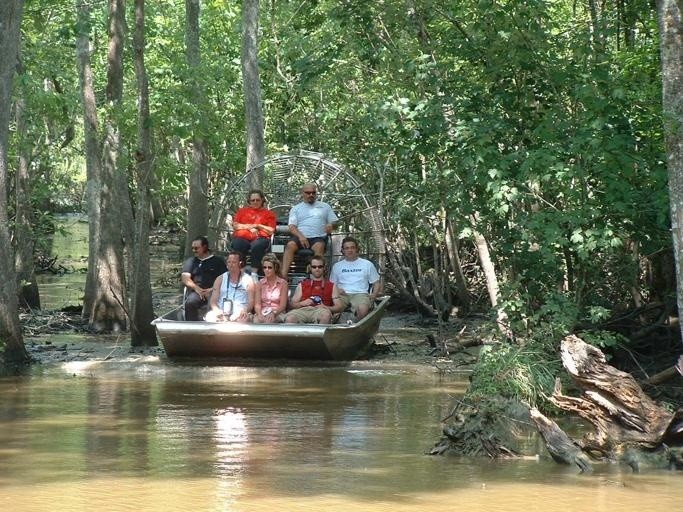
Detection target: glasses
<box><xmin>340</xmin><ymin>246</ymin><xmax>357</xmax><ymax>251</ymax></box>
<box><xmin>263</xmin><ymin>264</ymin><xmax>275</xmax><ymax>270</ymax></box>
<box><xmin>248</xmin><ymin>197</ymin><xmax>262</xmax><ymax>202</ymax></box>
<box><xmin>309</xmin><ymin>264</ymin><xmax>324</xmax><ymax>269</ymax></box>
<box><xmin>302</xmin><ymin>189</ymin><xmax>317</xmax><ymax>194</ymax></box>
<box><xmin>190</xmin><ymin>246</ymin><xmax>203</xmax><ymax>250</ymax></box>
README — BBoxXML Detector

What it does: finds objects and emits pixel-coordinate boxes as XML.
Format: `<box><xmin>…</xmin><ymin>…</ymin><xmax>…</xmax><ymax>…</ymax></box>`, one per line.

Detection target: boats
<box><xmin>149</xmin><ymin>149</ymin><xmax>391</xmax><ymax>363</ymax></box>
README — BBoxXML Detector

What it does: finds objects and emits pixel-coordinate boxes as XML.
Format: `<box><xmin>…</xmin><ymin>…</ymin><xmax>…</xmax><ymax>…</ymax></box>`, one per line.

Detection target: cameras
<box><xmin>312</xmin><ymin>296</ymin><xmax>321</xmax><ymax>303</ymax></box>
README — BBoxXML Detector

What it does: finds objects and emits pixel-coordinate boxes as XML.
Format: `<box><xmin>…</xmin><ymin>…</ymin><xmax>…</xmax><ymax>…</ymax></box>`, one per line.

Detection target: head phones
<box><xmin>238</xmin><ymin>253</ymin><xmax>246</xmax><ymax>268</ymax></box>
<box><xmin>306</xmin><ymin>262</ymin><xmax>329</xmax><ymax>274</ymax></box>
<box><xmin>247</xmin><ymin>195</ymin><xmax>265</xmax><ymax>204</ymax></box>
<box><xmin>267</xmin><ymin>255</ymin><xmax>279</xmax><ymax>273</ymax></box>
<box><xmin>341</xmin><ymin>245</ymin><xmax>359</xmax><ymax>255</ymax></box>
<box><xmin>200</xmin><ymin>237</ymin><xmax>208</xmax><ymax>253</ymax></box>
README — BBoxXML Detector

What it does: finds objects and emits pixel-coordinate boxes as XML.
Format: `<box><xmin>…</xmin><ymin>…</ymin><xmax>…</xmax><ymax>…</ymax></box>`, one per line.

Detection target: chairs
<box><xmin>246</xmin><ymin>234</ymin><xmax>275</xmax><ymax>275</ymax></box>
<box><xmin>295</xmin><ymin>237</ymin><xmax>329</xmax><ymax>277</ymax></box>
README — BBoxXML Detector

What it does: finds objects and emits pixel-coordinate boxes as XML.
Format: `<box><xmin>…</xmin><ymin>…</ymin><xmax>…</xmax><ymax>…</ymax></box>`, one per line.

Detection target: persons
<box><xmin>285</xmin><ymin>254</ymin><xmax>345</xmax><ymax>324</ymax></box>
<box><xmin>330</xmin><ymin>237</ymin><xmax>380</xmax><ymax>322</ymax></box>
<box><xmin>252</xmin><ymin>253</ymin><xmax>288</xmax><ymax>323</ymax></box>
<box><xmin>180</xmin><ymin>236</ymin><xmax>228</xmax><ymax>320</ymax></box>
<box><xmin>282</xmin><ymin>184</ymin><xmax>340</xmax><ymax>277</ymax></box>
<box><xmin>205</xmin><ymin>250</ymin><xmax>255</xmax><ymax>323</ymax></box>
<box><xmin>231</xmin><ymin>189</ymin><xmax>277</xmax><ymax>267</ymax></box>
